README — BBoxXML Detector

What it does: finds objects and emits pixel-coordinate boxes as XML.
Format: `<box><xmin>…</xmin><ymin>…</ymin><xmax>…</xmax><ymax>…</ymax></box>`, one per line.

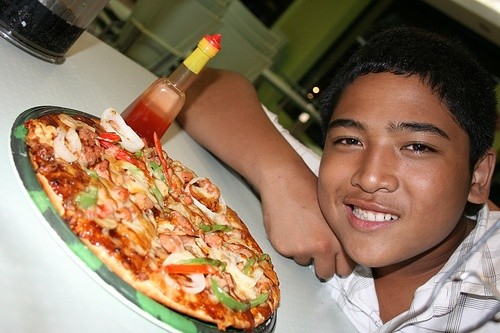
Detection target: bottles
<box><xmin>120</xmin><ymin>32</ymin><xmax>222</xmax><ymax>149</ymax></box>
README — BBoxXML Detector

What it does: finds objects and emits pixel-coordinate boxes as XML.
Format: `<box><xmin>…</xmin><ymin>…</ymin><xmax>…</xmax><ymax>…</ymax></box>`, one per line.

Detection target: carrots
<box><xmin>100</xmin><ymin>139</ymin><xmax>146</xmax><ymax>170</ymax></box>
<box><xmin>166</xmin><ymin>265</ymin><xmax>210</xmax><ymax>275</ymax></box>
<box><xmin>153</xmin><ymin>131</ymin><xmax>167</xmax><ymax>174</ymax></box>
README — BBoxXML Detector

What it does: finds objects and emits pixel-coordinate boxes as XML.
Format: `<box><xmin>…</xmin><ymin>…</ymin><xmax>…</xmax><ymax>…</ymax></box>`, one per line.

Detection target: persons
<box><xmin>175</xmin><ymin>24</ymin><xmax>500</xmax><ymax>332</ymax></box>
